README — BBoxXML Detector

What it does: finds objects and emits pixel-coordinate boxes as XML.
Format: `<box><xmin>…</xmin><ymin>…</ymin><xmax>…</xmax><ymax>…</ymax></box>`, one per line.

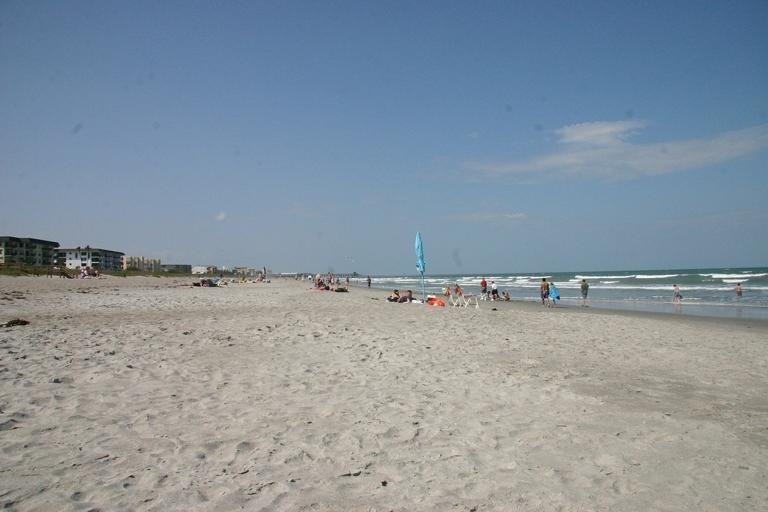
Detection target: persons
<box><xmin>735</xmin><ymin>283</ymin><xmax>742</xmax><ymax>296</ymax></box>
<box><xmin>581</xmin><ymin>279</ymin><xmax>589</xmax><ymax>307</ymax></box>
<box><xmin>547</xmin><ymin>282</ymin><xmax>560</xmax><ymax>305</ymax></box>
<box><xmin>199</xmin><ymin>272</ymin><xmax>269</xmax><ymax>287</ymax></box>
<box><xmin>672</xmin><ymin>284</ymin><xmax>681</xmax><ymax>304</ymax></box>
<box><xmin>392</xmin><ymin>289</ymin><xmax>400</xmax><ymax>301</ymax></box>
<box><xmin>674</xmin><ymin>302</ymin><xmax>682</xmax><ymax>312</ymax></box>
<box><xmin>479</xmin><ymin>277</ymin><xmax>510</xmax><ymax>301</ymax></box>
<box><xmin>293</xmin><ymin>270</ymin><xmax>372</xmax><ymax>292</ymax></box>
<box><xmin>540</xmin><ymin>278</ymin><xmax>551</xmax><ymax>308</ymax></box>
<box><xmin>396</xmin><ymin>290</ymin><xmax>417</xmax><ymax>303</ymax></box>
<box><xmin>79</xmin><ymin>265</ymin><xmax>101</xmax><ymax>279</ymax></box>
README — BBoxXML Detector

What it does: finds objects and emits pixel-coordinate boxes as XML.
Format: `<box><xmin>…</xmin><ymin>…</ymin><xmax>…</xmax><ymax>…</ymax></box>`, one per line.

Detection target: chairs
<box><xmin>449</xmin><ymin>293</ymin><xmax>479</xmax><ymax>308</ymax></box>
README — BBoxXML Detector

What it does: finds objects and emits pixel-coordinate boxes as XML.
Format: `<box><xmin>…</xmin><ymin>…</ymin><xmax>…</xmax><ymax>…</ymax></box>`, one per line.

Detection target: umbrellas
<box><xmin>414</xmin><ymin>231</ymin><xmax>427</xmax><ymax>301</ymax></box>
